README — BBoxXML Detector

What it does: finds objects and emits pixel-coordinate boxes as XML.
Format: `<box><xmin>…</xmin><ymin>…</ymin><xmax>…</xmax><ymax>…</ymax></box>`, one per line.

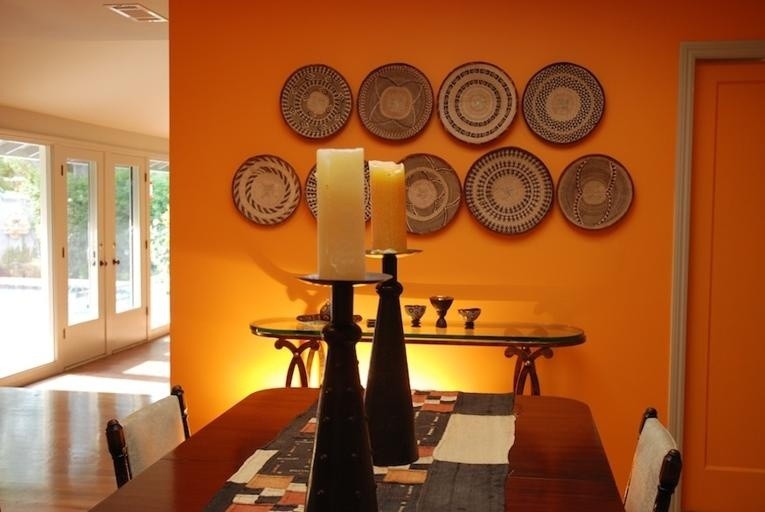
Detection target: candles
<box><xmin>366</xmin><ymin>159</ymin><xmax>409</xmax><ymax>251</ymax></box>
<box><xmin>315</xmin><ymin>150</ymin><xmax>370</xmax><ymax>280</ymax></box>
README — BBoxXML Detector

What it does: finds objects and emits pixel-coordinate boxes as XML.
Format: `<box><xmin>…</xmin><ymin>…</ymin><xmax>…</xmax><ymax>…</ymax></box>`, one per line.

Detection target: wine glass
<box><xmin>458</xmin><ymin>308</ymin><xmax>481</xmax><ymax>329</ymax></box>
<box><xmin>404</xmin><ymin>304</ymin><xmax>426</xmax><ymax>328</ymax></box>
<box><xmin>429</xmin><ymin>295</ymin><xmax>454</xmax><ymax>328</ymax></box>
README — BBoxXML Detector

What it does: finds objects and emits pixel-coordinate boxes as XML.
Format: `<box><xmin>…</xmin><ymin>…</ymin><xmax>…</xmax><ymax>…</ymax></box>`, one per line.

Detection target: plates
<box><xmin>394</xmin><ymin>151</ymin><xmax>463</xmax><ymax>236</ymax></box>
<box><xmin>231</xmin><ymin>154</ymin><xmax>302</xmax><ymax>226</ymax></box>
<box><xmin>436</xmin><ymin>60</ymin><xmax>519</xmax><ymax>146</ymax></box>
<box><xmin>555</xmin><ymin>152</ymin><xmax>635</xmax><ymax>231</ymax></box>
<box><xmin>356</xmin><ymin>62</ymin><xmax>436</xmax><ymax>141</ymax></box>
<box><xmin>463</xmin><ymin>145</ymin><xmax>556</xmax><ymax>236</ymax></box>
<box><xmin>304</xmin><ymin>157</ymin><xmax>372</xmax><ymax>224</ymax></box>
<box><xmin>279</xmin><ymin>63</ymin><xmax>353</xmax><ymax>140</ymax></box>
<box><xmin>520</xmin><ymin>61</ymin><xmax>606</xmax><ymax>146</ymax></box>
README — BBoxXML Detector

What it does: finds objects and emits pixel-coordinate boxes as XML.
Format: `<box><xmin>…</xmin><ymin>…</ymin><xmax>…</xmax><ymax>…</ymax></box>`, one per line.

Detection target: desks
<box><xmin>88</xmin><ymin>385</ymin><xmax>625</xmax><ymax>511</ymax></box>
<box><xmin>250</xmin><ymin>316</ymin><xmax>586</xmax><ymax>397</ymax></box>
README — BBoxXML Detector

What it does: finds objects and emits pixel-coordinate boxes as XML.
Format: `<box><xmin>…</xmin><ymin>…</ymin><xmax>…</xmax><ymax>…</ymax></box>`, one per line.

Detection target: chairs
<box><xmin>621</xmin><ymin>404</ymin><xmax>684</xmax><ymax>512</ymax></box>
<box><xmin>104</xmin><ymin>384</ymin><xmax>190</xmax><ymax>487</ymax></box>
